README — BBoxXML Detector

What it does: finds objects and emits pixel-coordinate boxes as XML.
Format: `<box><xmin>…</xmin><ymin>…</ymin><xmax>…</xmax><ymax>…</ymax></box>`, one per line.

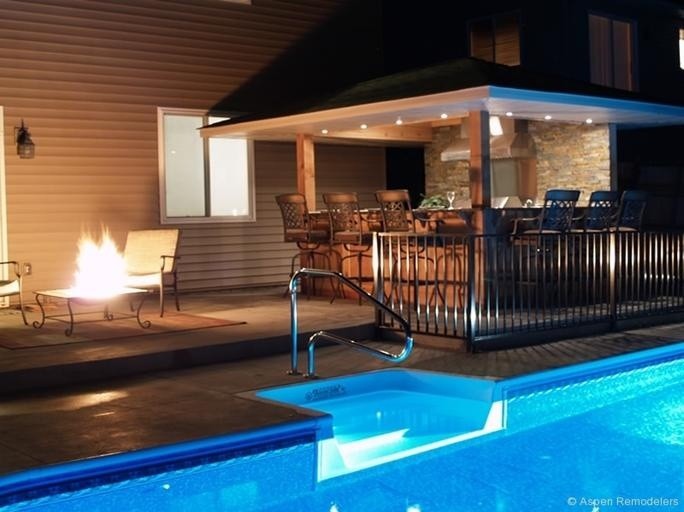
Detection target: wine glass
<box><xmin>447</xmin><ymin>192</ymin><xmax>455</xmax><ymax>209</ymax></box>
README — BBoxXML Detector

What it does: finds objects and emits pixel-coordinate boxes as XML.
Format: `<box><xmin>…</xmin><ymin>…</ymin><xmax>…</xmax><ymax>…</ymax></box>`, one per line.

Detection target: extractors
<box><xmin>440</xmin><ymin>114</ymin><xmax>536</xmax><ymax>162</ymax></box>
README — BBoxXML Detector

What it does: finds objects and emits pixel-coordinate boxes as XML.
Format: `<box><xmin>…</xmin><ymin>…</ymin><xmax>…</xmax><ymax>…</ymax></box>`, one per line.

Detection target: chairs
<box><xmin>0</xmin><ymin>258</ymin><xmax>36</xmax><ymax>330</ymax></box>
<box><xmin>122</xmin><ymin>228</ymin><xmax>182</xmax><ymax>318</ymax></box>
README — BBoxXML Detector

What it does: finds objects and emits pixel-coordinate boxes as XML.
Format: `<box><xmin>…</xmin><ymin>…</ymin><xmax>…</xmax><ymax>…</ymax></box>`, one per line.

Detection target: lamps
<box><xmin>13</xmin><ymin>116</ymin><xmax>36</xmax><ymax>160</ymax></box>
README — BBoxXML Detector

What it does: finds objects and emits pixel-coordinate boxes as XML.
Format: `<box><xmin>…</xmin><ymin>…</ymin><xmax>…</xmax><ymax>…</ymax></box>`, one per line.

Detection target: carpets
<box><xmin>0</xmin><ymin>298</ymin><xmax>246</xmax><ymax>350</ymax></box>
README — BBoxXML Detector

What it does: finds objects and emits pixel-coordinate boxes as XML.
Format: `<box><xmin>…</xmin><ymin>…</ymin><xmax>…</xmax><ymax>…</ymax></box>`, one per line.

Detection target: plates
<box><xmin>419</xmin><ymin>206</ymin><xmax>445</xmax><ymax>210</ymax></box>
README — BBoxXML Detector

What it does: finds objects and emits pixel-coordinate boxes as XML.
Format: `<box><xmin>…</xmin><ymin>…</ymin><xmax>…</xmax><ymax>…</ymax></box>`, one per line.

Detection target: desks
<box><xmin>32</xmin><ymin>284</ymin><xmax>152</xmax><ymax>337</ymax></box>
